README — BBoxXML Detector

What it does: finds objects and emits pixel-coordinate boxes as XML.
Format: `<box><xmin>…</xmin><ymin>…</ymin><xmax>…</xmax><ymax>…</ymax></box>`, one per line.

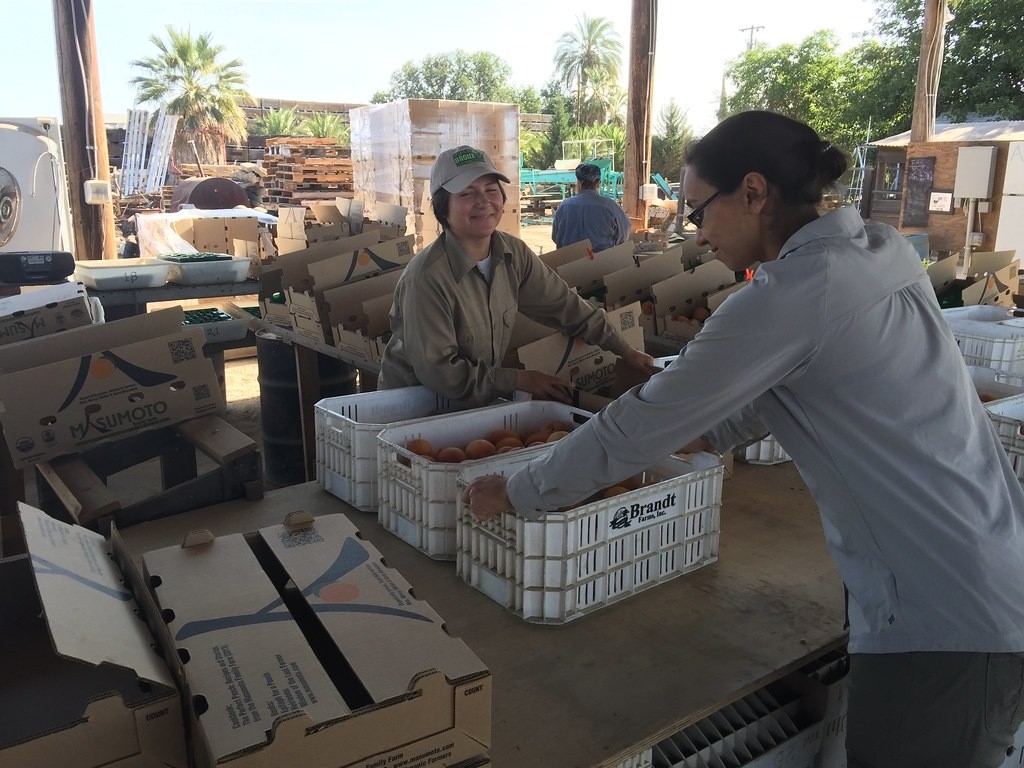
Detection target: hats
<box><xmin>575</xmin><ymin>163</ymin><xmax>601</xmax><ymax>182</ymax></box>
<box><xmin>429</xmin><ymin>144</ymin><xmax>511</xmax><ymax>197</ymax></box>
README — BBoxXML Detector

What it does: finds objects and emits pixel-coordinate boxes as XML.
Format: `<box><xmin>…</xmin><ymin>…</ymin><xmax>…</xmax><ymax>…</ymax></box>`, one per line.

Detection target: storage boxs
<box><xmin>1</xmin><ymin>94</ymin><xmax>1023</xmax><ymax>768</ymax></box>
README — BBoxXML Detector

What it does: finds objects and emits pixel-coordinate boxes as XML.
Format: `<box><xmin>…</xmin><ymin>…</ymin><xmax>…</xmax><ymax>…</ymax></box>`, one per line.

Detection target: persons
<box><xmin>373</xmin><ymin>145</ymin><xmax>655</xmax><ymax>403</ymax></box>
<box><xmin>459</xmin><ymin>111</ymin><xmax>1024</xmax><ymax>768</ymax></box>
<box><xmin>550</xmin><ymin>162</ymin><xmax>632</xmax><ymax>253</ymax></box>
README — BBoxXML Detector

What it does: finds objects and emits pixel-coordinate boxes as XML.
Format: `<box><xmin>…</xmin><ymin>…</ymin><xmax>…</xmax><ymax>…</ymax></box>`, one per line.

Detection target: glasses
<box><xmin>687</xmin><ymin>176</ymin><xmax>741</xmax><ymax>229</ymax></box>
